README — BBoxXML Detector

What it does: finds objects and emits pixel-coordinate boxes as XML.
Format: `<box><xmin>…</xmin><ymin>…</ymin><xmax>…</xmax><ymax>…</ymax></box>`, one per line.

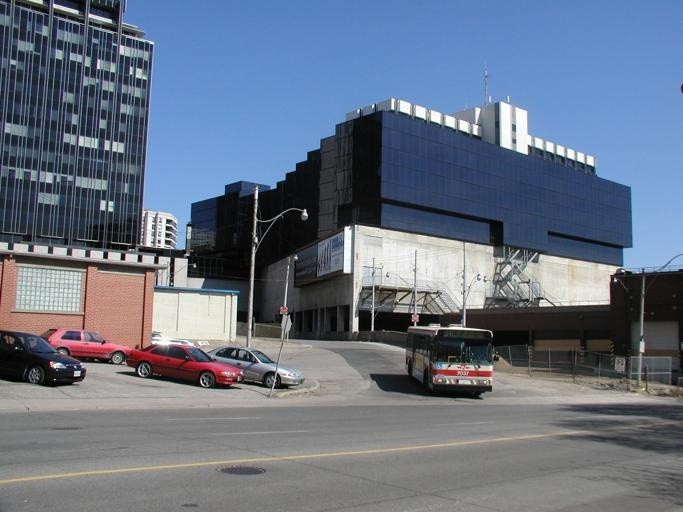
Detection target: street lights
<box><xmin>386</xmin><ymin>249</ymin><xmax>418</xmax><ymax>327</ymax></box>
<box><xmin>283</xmin><ymin>254</ymin><xmax>299</xmax><ymax>308</ymax></box>
<box><xmin>462</xmin><ymin>241</ymin><xmax>488</xmax><ymax>325</ymax></box>
<box><xmin>247</xmin><ymin>185</ymin><xmax>308</xmax><ymax>346</ymax></box>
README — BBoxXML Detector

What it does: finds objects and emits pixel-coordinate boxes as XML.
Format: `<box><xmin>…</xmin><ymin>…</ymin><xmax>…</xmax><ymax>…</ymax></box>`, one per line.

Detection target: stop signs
<box><xmin>280</xmin><ymin>306</ymin><xmax>288</xmax><ymax>315</ymax></box>
<box><xmin>412</xmin><ymin>315</ymin><xmax>419</xmax><ymax>322</ymax></box>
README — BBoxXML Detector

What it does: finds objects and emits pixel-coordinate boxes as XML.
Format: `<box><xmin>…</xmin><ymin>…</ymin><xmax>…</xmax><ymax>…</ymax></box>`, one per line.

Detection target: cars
<box><xmin>207</xmin><ymin>346</ymin><xmax>304</xmax><ymax>388</ymax></box>
<box><xmin>0</xmin><ymin>330</ymin><xmax>86</xmax><ymax>385</ymax></box>
<box><xmin>126</xmin><ymin>343</ymin><xmax>244</xmax><ymax>389</ymax></box>
<box><xmin>42</xmin><ymin>328</ymin><xmax>131</xmax><ymax>364</ymax></box>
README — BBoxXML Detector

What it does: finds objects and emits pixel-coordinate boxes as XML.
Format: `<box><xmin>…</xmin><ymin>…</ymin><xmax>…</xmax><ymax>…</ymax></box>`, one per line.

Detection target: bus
<box><xmin>405</xmin><ymin>324</ymin><xmax>494</xmax><ymax>397</ymax></box>
